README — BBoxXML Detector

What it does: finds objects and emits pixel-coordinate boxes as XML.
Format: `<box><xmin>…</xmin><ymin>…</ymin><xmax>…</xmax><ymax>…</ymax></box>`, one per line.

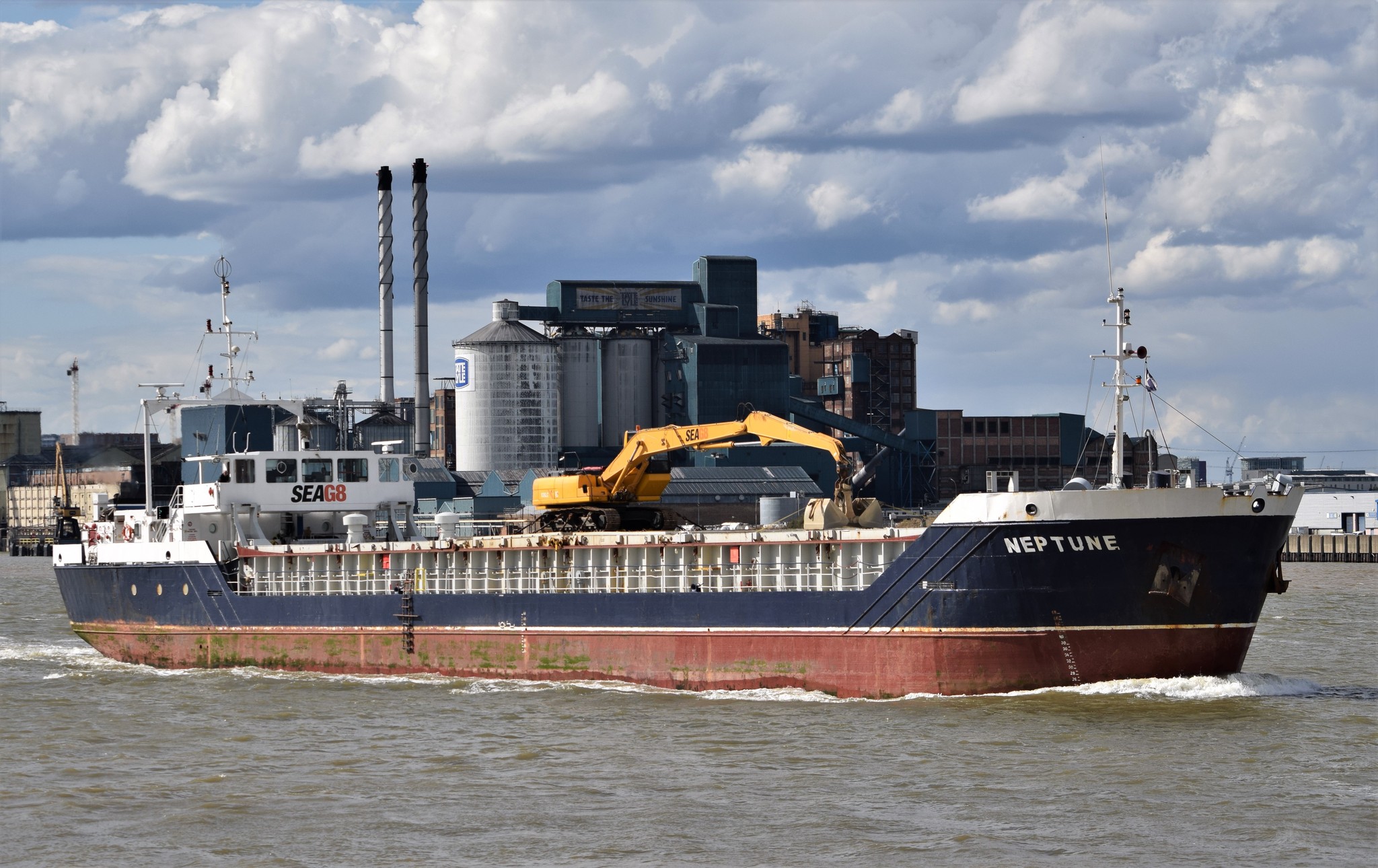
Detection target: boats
<box><xmin>52</xmin><ymin>159</ymin><xmax>1310</xmax><ymax>704</ymax></box>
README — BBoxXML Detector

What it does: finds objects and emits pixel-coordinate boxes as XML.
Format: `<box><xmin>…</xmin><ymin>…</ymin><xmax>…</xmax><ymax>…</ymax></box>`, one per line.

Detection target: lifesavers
<box><xmin>122</xmin><ymin>524</ymin><xmax>132</xmax><ymax>540</ymax></box>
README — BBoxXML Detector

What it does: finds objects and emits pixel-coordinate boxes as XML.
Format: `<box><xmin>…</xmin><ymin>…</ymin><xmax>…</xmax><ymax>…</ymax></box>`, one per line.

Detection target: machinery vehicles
<box><xmin>528</xmin><ymin>412</ymin><xmax>892</xmax><ymax>530</ymax></box>
<box><xmin>46</xmin><ymin>441</ymin><xmax>85</xmax><ymax>542</ymax></box>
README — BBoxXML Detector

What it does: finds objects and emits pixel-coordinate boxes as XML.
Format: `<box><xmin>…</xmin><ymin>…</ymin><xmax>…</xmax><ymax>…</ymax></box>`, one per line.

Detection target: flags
<box><xmin>1145</xmin><ymin>369</ymin><xmax>1158</xmax><ymax>392</ymax></box>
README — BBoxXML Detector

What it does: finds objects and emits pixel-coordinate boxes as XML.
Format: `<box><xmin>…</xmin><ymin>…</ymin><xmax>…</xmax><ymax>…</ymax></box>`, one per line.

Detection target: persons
<box><xmin>217</xmin><ymin>470</ymin><xmax>229</xmax><ymax>482</ymax></box>
